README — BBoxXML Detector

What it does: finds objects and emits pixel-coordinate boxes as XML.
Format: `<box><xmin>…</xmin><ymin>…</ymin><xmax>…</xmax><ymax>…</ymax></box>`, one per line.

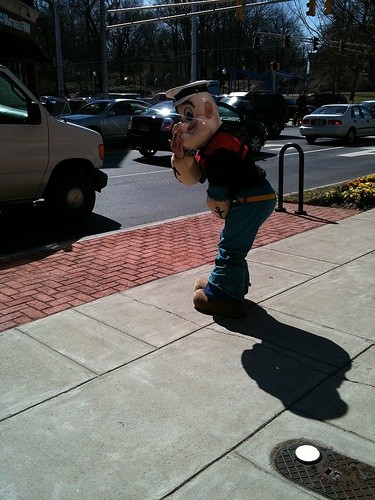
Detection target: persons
<box><xmin>162</xmin><ymin>79</ymin><xmax>276</xmax><ymax>318</ymax></box>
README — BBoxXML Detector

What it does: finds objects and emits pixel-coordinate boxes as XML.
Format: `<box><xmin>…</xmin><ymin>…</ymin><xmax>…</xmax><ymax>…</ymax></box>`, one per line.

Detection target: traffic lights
<box><xmin>284</xmin><ymin>34</ymin><xmax>290</xmax><ymax>47</ymax></box>
<box><xmin>273</xmin><ymin>62</ymin><xmax>277</xmax><ymax>70</ymax></box>
<box><xmin>313</xmin><ymin>35</ymin><xmax>319</xmax><ymax>50</ymax></box>
<box><xmin>306</xmin><ymin>0</ymin><xmax>315</xmax><ymax>16</ymax></box>
<box><xmin>277</xmin><ymin>63</ymin><xmax>280</xmax><ymax>71</ymax></box>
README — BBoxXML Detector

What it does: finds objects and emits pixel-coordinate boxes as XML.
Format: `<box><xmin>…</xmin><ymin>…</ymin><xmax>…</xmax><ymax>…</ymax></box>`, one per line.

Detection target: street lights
<box><xmin>222</xmin><ymin>68</ymin><xmax>226</xmax><ymax>94</ymax></box>
<box><xmin>93</xmin><ymin>69</ymin><xmax>96</xmax><ymax>97</ymax></box>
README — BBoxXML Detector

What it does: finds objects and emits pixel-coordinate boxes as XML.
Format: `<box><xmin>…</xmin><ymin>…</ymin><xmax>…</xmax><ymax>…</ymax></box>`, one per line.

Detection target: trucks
<box><xmin>0</xmin><ymin>0</ymin><xmax>108</xmax><ymax>223</ymax></box>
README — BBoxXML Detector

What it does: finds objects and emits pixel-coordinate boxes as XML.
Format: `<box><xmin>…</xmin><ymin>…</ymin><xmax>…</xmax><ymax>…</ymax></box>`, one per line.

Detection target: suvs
<box><xmin>216</xmin><ymin>91</ymin><xmax>289</xmax><ymax>139</ymax></box>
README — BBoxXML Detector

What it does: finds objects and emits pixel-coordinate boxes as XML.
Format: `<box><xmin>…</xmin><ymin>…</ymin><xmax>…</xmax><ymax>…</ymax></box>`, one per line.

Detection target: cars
<box><xmin>299</xmin><ymin>104</ymin><xmax>375</xmax><ymax>145</ymax></box>
<box><xmin>301</xmin><ymin>94</ymin><xmax>348</xmax><ymax>116</ymax></box>
<box><xmin>128</xmin><ymin>99</ymin><xmax>268</xmax><ymax>156</ymax></box>
<box><xmin>39</xmin><ymin>92</ymin><xmax>169</xmax><ymax>146</ymax></box>
